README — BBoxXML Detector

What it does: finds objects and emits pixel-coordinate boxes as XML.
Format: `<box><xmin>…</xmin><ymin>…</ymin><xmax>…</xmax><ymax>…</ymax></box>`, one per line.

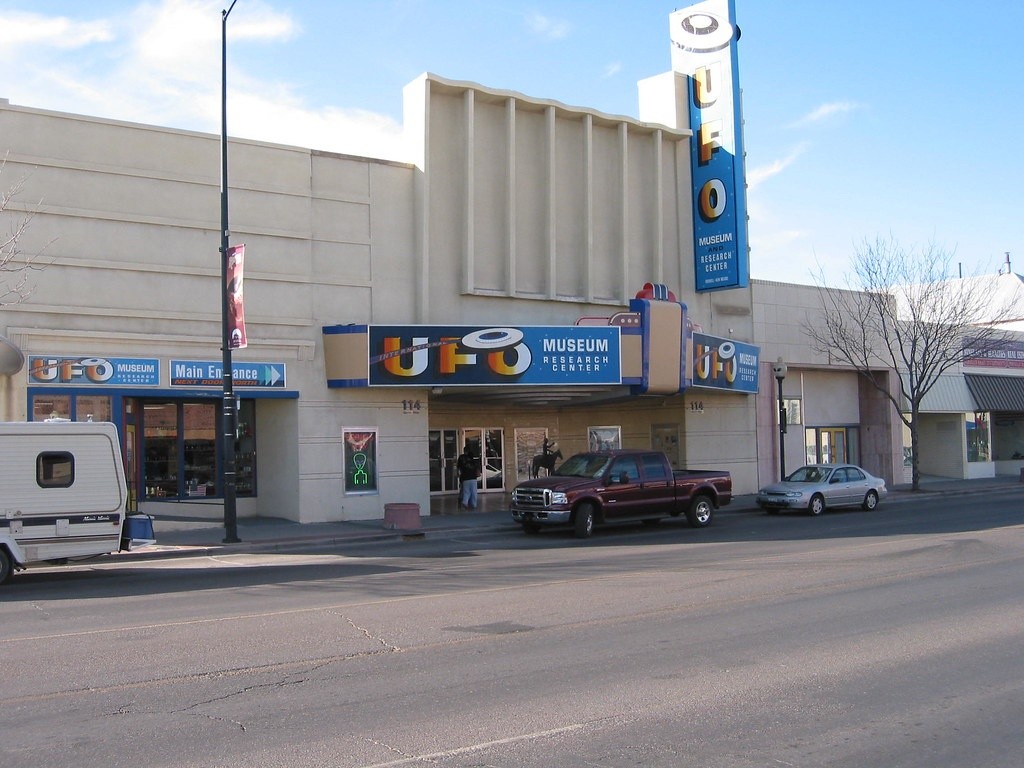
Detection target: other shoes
<box><xmin>461</xmin><ymin>502</ymin><xmax>467</xmax><ymax>509</ymax></box>
<box><xmin>472</xmin><ymin>507</ymin><xmax>478</xmax><ymax>511</ymax></box>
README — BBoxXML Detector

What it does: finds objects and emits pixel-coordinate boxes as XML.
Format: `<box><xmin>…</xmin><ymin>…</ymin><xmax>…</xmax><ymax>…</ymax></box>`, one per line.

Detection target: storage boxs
<box><xmin>126</xmin><ymin>514</ymin><xmax>155</xmax><ymax>541</ymax></box>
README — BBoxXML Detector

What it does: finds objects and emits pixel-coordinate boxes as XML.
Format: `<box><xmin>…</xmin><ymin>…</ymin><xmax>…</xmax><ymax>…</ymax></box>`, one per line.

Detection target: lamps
<box><xmin>432</xmin><ymin>386</ymin><xmax>444</xmax><ymax>395</ymax></box>
<box><xmin>401</xmin><ymin>399</ymin><xmax>422</xmax><ymax>415</ymax></box>
<box><xmin>690</xmin><ymin>400</ymin><xmax>704</xmax><ymax>415</ymax></box>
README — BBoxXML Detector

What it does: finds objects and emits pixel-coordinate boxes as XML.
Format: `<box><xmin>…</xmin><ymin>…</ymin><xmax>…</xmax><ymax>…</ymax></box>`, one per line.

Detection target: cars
<box><xmin>756</xmin><ymin>464</ymin><xmax>889</xmax><ymax>516</ymax></box>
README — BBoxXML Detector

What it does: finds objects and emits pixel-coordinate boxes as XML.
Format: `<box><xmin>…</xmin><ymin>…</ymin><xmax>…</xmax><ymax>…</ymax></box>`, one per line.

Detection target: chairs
<box><xmin>623</xmin><ymin>465</ymin><xmax>639</xmax><ymax>480</ymax></box>
<box><xmin>586</xmin><ymin>459</ymin><xmax>605</xmax><ymax>476</ymax></box>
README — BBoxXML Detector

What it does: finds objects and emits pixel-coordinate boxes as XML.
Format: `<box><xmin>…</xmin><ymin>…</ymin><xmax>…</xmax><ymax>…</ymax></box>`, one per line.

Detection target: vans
<box><xmin>0</xmin><ymin>413</ymin><xmax>158</xmax><ymax>585</ymax></box>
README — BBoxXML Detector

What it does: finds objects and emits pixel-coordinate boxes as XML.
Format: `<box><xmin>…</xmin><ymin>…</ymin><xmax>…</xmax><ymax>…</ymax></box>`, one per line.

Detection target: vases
<box><xmin>383</xmin><ymin>503</ymin><xmax>422</xmax><ymax>530</ymax></box>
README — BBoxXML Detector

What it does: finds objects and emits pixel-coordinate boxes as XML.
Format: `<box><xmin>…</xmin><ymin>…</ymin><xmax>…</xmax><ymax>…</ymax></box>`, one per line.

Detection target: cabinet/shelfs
<box><xmin>146</xmin><ymin>434</ymin><xmax>255</xmax><ymax>496</ymax></box>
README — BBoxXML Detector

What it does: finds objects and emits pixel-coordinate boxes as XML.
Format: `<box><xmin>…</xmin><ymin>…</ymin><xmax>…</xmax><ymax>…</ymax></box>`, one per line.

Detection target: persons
<box><xmin>457</xmin><ymin>447</ymin><xmax>481</xmax><ymax>510</ymax></box>
<box><xmin>543</xmin><ymin>437</ymin><xmax>555</xmax><ymax>455</ymax></box>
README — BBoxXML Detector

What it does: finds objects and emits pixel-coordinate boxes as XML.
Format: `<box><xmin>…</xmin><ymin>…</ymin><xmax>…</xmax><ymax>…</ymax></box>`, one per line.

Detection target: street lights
<box><xmin>773</xmin><ymin>356</ymin><xmax>787</xmax><ymax>482</ymax></box>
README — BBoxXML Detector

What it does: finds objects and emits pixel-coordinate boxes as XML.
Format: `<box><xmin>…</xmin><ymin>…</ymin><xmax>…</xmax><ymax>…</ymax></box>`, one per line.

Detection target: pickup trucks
<box><xmin>510</xmin><ymin>449</ymin><xmax>735</xmax><ymax>539</ymax></box>
